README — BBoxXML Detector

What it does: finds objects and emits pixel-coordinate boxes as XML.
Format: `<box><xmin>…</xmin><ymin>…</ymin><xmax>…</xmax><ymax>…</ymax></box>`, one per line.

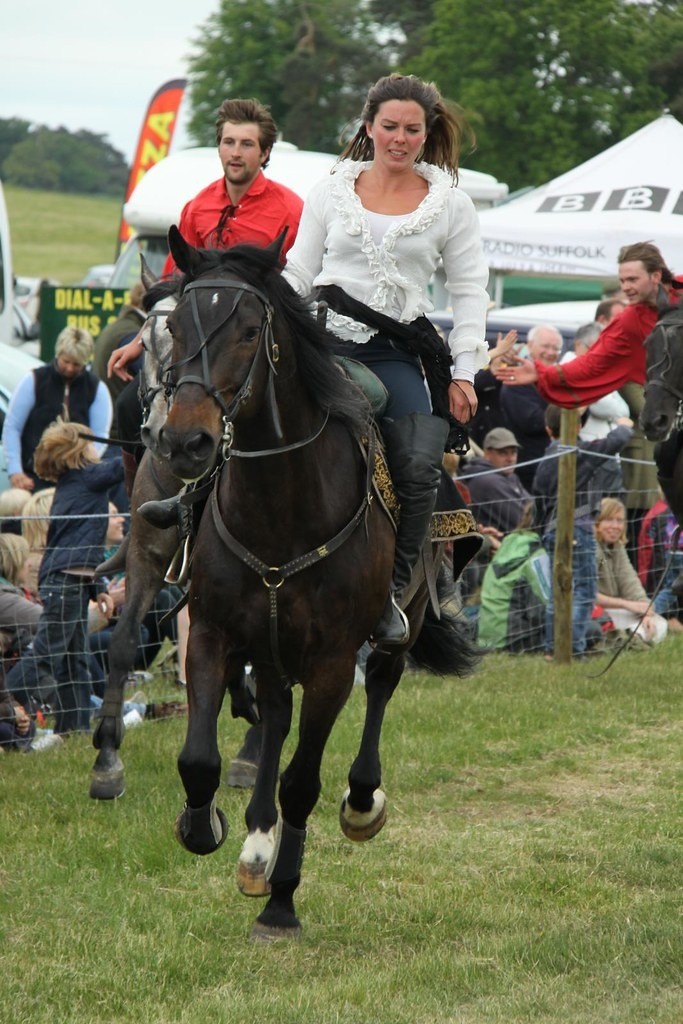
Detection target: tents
<box><xmin>429</xmin><ymin>110</ymin><xmax>683</xmax><ymax>307</ymax></box>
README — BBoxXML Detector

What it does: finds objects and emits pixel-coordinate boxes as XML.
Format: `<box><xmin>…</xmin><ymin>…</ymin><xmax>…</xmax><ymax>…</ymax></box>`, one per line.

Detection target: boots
<box><xmin>373</xmin><ymin>414</ymin><xmax>451</xmax><ymax>644</ymax></box>
<box><xmin>656</xmin><ymin>471</ymin><xmax>683</xmax><ymax>594</ymax></box>
<box><xmin>136</xmin><ymin>495</ymin><xmax>181</xmax><ymax>529</ymax></box>
<box><xmin>95</xmin><ymin>445</ymin><xmax>142</xmax><ymax>575</ymax></box>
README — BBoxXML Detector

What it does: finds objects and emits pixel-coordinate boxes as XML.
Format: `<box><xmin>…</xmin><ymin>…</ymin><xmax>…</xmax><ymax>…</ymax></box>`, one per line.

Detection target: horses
<box><xmin>640</xmin><ymin>286</ymin><xmax>683</xmax><ymax>523</ymax></box>
<box><xmin>74</xmin><ymin>224</ymin><xmax>498</xmax><ymax>939</ymax></box>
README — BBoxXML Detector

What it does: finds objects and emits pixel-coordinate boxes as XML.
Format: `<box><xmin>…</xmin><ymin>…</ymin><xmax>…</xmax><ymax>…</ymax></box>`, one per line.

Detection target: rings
<box><xmin>509</xmin><ymin>377</ymin><xmax>515</xmax><ymax>381</ymax></box>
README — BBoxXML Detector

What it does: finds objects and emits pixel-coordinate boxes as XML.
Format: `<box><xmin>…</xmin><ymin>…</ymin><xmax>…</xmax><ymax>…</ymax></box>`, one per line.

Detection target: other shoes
<box><xmin>35</xmin><ymin>728</ymin><xmax>53</xmax><ymax>735</ymax></box>
<box><xmin>668</xmin><ymin>617</ymin><xmax>683</xmax><ymax>631</ymax></box>
<box><xmin>129</xmin><ymin>691</ymin><xmax>148</xmax><ymax>705</ymax></box>
<box><xmin>30</xmin><ymin>734</ymin><xmax>63</xmax><ymax>752</ymax></box>
<box><xmin>131</xmin><ymin>671</ymin><xmax>153</xmax><ymax>682</ymax></box>
<box><xmin>154</xmin><ymin>702</ymin><xmax>183</xmax><ymax>717</ymax></box>
<box><xmin>175</xmin><ymin>679</ymin><xmax>186</xmax><ymax>685</ymax></box>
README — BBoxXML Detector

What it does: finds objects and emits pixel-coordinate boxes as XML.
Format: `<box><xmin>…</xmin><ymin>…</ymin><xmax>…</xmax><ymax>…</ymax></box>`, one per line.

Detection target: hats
<box><xmin>483</xmin><ymin>427</ymin><xmax>520</xmax><ymax>449</ymax></box>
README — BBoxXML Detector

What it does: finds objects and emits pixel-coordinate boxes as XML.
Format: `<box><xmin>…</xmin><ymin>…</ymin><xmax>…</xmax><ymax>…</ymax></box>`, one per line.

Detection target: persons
<box><xmin>276</xmin><ymin>69</ymin><xmax>488</xmax><ymax>649</ymax></box>
<box><xmin>94</xmin><ymin>99</ymin><xmax>304</xmax><ymax>576</ymax></box>
<box><xmin>494</xmin><ymin>244</ymin><xmax>682</xmax><ymax>524</ymax></box>
<box><xmin>0</xmin><ymin>283</ymin><xmax>683</xmax><ymax>756</ymax></box>
